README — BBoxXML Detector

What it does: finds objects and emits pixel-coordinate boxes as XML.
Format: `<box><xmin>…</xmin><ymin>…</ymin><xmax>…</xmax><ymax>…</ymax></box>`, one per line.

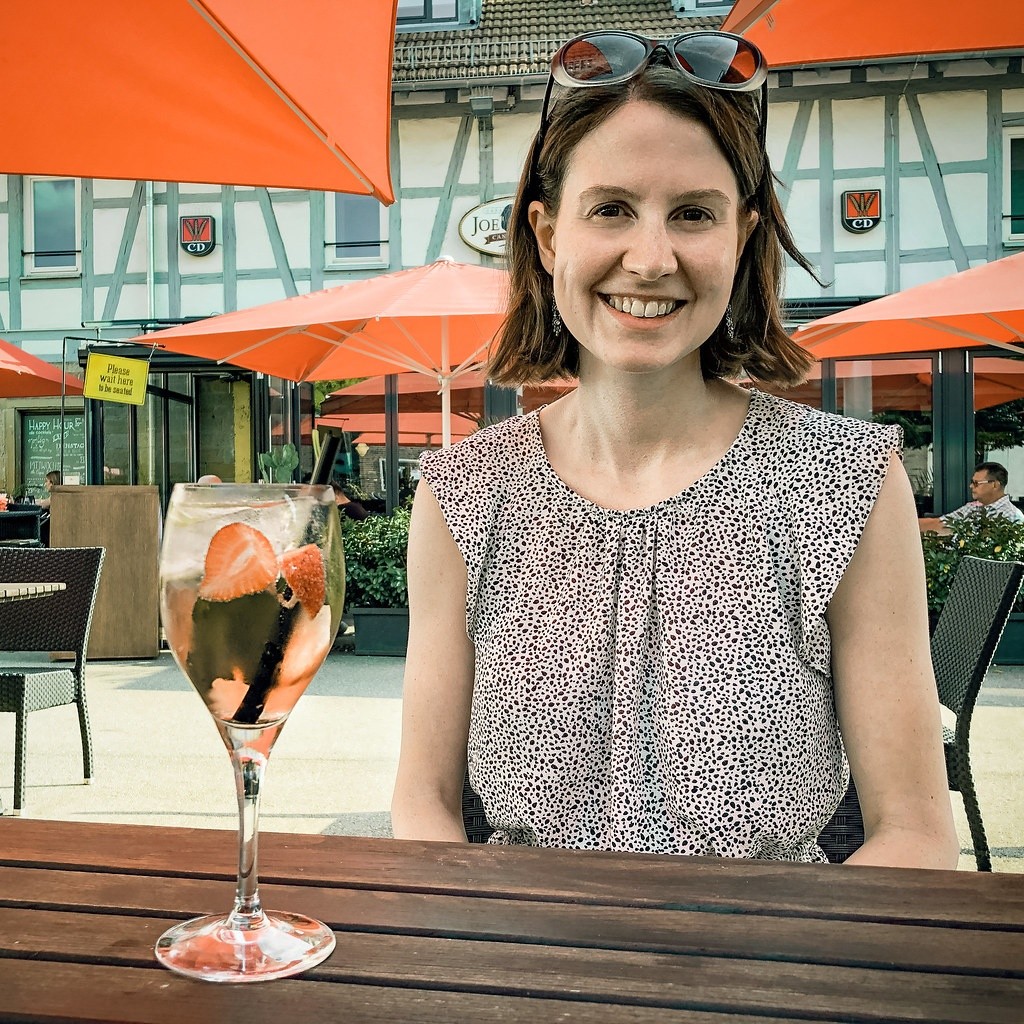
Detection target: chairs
<box><xmin>930</xmin><ymin>555</ymin><xmax>1024</xmax><ymax>872</ymax></box>
<box><xmin>0</xmin><ymin>547</ymin><xmax>106</xmax><ymax>809</ymax></box>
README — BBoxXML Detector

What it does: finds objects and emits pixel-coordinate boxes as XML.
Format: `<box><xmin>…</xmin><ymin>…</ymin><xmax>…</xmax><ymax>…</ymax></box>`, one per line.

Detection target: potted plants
<box><xmin>342</xmin><ymin>494</ymin><xmax>414</xmax><ymax>656</ymax></box>
<box><xmin>920</xmin><ymin>504</ymin><xmax>1024</xmax><ymax>666</ymax></box>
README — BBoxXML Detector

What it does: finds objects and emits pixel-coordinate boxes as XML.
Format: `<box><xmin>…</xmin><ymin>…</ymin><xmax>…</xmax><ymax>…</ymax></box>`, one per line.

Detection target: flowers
<box><xmin>0</xmin><ymin>493</ymin><xmax>9</xmax><ymax>512</ymax></box>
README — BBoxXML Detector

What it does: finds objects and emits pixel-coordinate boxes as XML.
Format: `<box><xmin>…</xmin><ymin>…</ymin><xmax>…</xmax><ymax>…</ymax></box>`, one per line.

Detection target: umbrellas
<box><xmin>269</xmin><ymin>366</ymin><xmax>579</xmax><ymax>449</ymax></box>
<box><xmin>0</xmin><ymin>0</ymin><xmax>398</xmax><ymax>209</ymax></box>
<box><xmin>0</xmin><ymin>338</ymin><xmax>83</xmax><ymax>398</ymax></box>
<box><xmin>786</xmin><ymin>251</ymin><xmax>1024</xmax><ymax>359</ymax></box>
<box><xmin>780</xmin><ymin>357</ymin><xmax>1024</xmax><ymax>412</ymax></box>
<box><xmin>133</xmin><ymin>255</ymin><xmax>576</xmax><ymax>445</ymax></box>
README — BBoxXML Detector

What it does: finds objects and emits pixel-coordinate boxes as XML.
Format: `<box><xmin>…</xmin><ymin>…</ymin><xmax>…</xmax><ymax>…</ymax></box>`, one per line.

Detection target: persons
<box><xmin>39</xmin><ymin>471</ymin><xmax>61</xmax><ymax>509</ymax></box>
<box><xmin>392</xmin><ymin>28</ymin><xmax>960</xmax><ymax>869</ymax></box>
<box><xmin>198</xmin><ymin>475</ymin><xmax>222</xmax><ymax>483</ymax></box>
<box><xmin>918</xmin><ymin>462</ymin><xmax>1024</xmax><ymax>553</ymax></box>
<box><xmin>301</xmin><ymin>474</ymin><xmax>369</xmax><ymax>636</ymax></box>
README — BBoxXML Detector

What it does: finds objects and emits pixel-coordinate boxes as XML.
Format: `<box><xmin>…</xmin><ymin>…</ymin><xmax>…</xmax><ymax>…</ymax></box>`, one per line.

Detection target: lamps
<box><xmin>469</xmin><ymin>96</ymin><xmax>494</xmax><ymax>115</ymax></box>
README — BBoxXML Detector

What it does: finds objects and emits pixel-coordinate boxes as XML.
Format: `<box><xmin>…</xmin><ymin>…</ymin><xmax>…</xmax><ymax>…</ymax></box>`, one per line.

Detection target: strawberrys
<box><xmin>282</xmin><ymin>545</ymin><xmax>325</xmax><ymax>620</ymax></box>
<box><xmin>201</xmin><ymin>522</ymin><xmax>279</xmax><ymax>602</ymax></box>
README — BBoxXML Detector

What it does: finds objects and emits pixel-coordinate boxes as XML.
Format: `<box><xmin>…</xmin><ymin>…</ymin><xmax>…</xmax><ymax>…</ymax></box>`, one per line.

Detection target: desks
<box><xmin>0</xmin><ymin>582</ymin><xmax>68</xmax><ymax>603</ymax></box>
<box><xmin>1</xmin><ymin>817</ymin><xmax>1023</xmax><ymax>1024</ymax></box>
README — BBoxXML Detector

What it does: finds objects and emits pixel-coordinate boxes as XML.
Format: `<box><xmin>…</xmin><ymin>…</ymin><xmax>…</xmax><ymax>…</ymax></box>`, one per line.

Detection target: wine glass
<box><xmin>152</xmin><ymin>478</ymin><xmax>346</xmax><ymax>983</ymax></box>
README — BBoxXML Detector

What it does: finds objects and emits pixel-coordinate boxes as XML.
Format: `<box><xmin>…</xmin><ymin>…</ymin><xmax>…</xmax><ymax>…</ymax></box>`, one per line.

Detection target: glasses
<box><xmin>542</xmin><ymin>29</ymin><xmax>770</xmax><ymax>129</ymax></box>
<box><xmin>971</xmin><ymin>479</ymin><xmax>995</xmax><ymax>488</ymax></box>
